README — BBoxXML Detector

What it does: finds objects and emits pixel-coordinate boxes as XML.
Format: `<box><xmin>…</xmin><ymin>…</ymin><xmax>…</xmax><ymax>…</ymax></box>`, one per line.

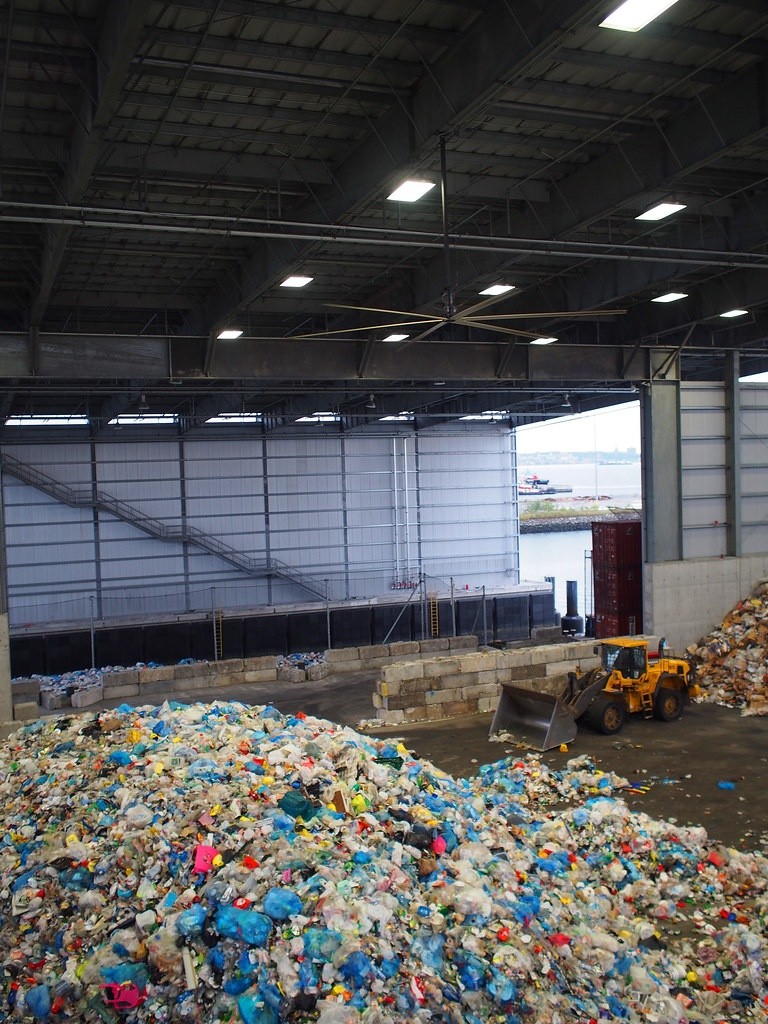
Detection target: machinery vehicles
<box><xmin>487</xmin><ymin>635</ymin><xmax>701</xmax><ymax>752</ymax></box>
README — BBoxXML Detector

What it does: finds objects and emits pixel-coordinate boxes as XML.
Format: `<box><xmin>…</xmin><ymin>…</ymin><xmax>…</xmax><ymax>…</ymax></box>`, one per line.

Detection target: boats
<box><xmin>517</xmin><ymin>477</ymin><xmax>573</xmax><ymax>495</ymax></box>
